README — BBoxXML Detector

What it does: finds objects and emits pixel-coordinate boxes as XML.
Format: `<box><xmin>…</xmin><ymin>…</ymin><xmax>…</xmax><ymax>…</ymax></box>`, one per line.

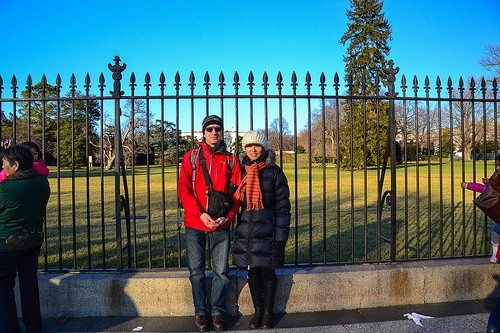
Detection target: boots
<box><xmin>490</xmin><ymin>243</ymin><xmax>499</xmax><ymax>263</ymax></box>
<box><xmin>262</xmin><ymin>278</ymin><xmax>277</xmax><ymax>329</ymax></box>
<box><xmin>247</xmin><ymin>278</ymin><xmax>264</xmax><ymax>329</ymax></box>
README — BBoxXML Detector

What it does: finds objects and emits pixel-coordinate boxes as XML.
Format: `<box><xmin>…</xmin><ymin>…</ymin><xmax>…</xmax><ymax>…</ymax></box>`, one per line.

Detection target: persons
<box><xmin>460</xmin><ymin>154</ymin><xmax>500</xmax><ymax>333</ymax></box>
<box><xmin>0</xmin><ymin>144</ymin><xmax>51</xmax><ymax>333</ymax></box>
<box><xmin>227</xmin><ymin>130</ymin><xmax>291</xmax><ymax>329</ymax></box>
<box><xmin>0</xmin><ymin>141</ymin><xmax>49</xmax><ymax>183</ymax></box>
<box><xmin>178</xmin><ymin>115</ymin><xmax>243</xmax><ymax>332</ymax></box>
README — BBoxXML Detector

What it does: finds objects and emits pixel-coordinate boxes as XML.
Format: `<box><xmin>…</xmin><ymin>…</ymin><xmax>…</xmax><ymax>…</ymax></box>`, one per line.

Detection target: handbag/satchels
<box><xmin>206</xmin><ymin>190</ymin><xmax>234</xmax><ymax>218</ymax></box>
<box><xmin>473</xmin><ymin>170</ymin><xmax>500</xmax><ymax>225</ymax></box>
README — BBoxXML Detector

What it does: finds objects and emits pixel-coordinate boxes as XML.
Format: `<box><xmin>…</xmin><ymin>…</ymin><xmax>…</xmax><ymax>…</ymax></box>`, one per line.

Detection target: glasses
<box><xmin>205</xmin><ymin>127</ymin><xmax>222</xmax><ymax>132</ymax></box>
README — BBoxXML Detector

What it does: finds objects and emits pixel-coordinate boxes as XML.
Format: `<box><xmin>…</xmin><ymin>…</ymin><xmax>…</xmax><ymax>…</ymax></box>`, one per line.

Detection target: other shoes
<box><xmin>195</xmin><ymin>315</ymin><xmax>209</xmax><ymax>331</ymax></box>
<box><xmin>487</xmin><ymin>326</ymin><xmax>500</xmax><ymax>333</ymax></box>
<box><xmin>211</xmin><ymin>314</ymin><xmax>226</xmax><ymax>331</ymax></box>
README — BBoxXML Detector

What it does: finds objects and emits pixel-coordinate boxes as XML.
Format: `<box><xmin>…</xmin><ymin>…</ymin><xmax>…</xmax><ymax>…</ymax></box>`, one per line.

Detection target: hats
<box><xmin>202</xmin><ymin>115</ymin><xmax>223</xmax><ymax>133</ymax></box>
<box><xmin>242</xmin><ymin>129</ymin><xmax>268</xmax><ymax>151</ymax></box>
